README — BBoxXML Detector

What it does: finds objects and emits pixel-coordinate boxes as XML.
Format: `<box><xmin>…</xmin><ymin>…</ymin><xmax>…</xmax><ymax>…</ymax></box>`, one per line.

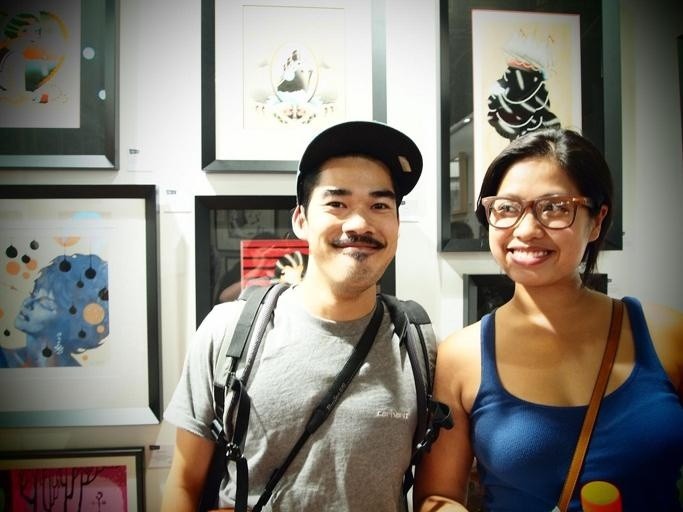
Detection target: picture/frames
<box><xmin>201</xmin><ymin>0</ymin><xmax>387</xmax><ymax>172</ymax></box>
<box><xmin>224</xmin><ymin>256</ymin><xmax>241</xmax><ymax>272</ymax></box>
<box><xmin>215</xmin><ymin>210</ymin><xmax>278</xmax><ymax>250</ymax></box>
<box><xmin>195</xmin><ymin>195</ymin><xmax>396</xmax><ymax>330</ymax></box>
<box><xmin>463</xmin><ymin>274</ymin><xmax>607</xmax><ymax>327</ymax></box>
<box><xmin>439</xmin><ymin>0</ymin><xmax>623</xmax><ymax>251</ymax></box>
<box><xmin>0</xmin><ymin>185</ymin><xmax>163</xmax><ymax>429</ymax></box>
<box><xmin>0</xmin><ymin>447</ymin><xmax>146</xmax><ymax>512</ymax></box>
<box><xmin>0</xmin><ymin>0</ymin><xmax>119</xmax><ymax>170</ymax></box>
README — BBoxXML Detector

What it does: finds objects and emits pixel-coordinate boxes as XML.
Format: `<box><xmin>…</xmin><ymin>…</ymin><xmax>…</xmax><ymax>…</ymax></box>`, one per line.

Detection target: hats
<box><xmin>296</xmin><ymin>122</ymin><xmax>423</xmax><ymax>202</ymax></box>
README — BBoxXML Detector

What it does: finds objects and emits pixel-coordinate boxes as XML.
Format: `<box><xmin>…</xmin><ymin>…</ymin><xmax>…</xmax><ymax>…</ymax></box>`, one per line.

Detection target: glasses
<box><xmin>480</xmin><ymin>193</ymin><xmax>591</xmax><ymax>231</ymax></box>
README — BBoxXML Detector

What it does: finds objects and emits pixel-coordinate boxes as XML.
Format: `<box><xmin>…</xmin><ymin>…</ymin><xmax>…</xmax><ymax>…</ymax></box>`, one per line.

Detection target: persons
<box><xmin>405</xmin><ymin>125</ymin><xmax>681</xmax><ymax>511</ymax></box>
<box><xmin>155</xmin><ymin>119</ymin><xmax>454</xmax><ymax>511</ymax></box>
<box><xmin>0</xmin><ymin>253</ymin><xmax>109</xmax><ymax>369</ymax></box>
<box><xmin>211</xmin><ymin>233</ymin><xmax>282</xmax><ymax>305</ymax></box>
